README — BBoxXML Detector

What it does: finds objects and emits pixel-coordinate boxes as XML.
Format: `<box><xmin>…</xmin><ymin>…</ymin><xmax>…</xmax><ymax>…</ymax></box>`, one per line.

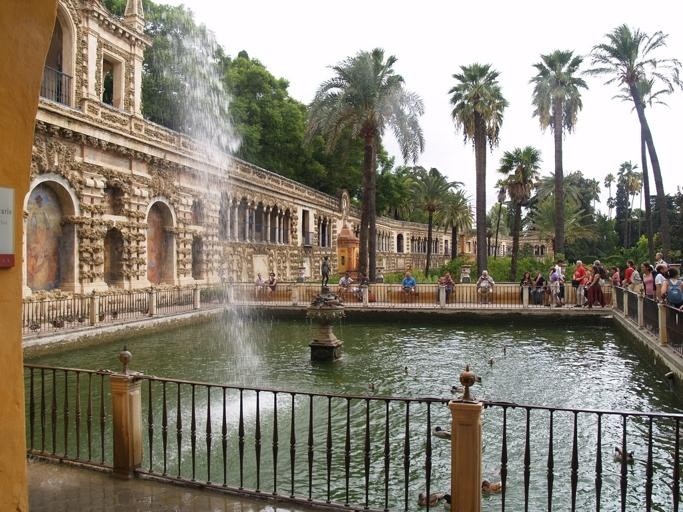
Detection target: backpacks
<box><xmin>667</xmin><ymin>279</ymin><xmax>683</xmax><ymax>305</ymax></box>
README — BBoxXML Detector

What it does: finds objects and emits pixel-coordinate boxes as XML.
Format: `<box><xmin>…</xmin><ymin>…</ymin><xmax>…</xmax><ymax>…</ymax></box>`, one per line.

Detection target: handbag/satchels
<box><xmin>572</xmin><ymin>280</ymin><xmax>579</xmax><ymax>288</ymax></box>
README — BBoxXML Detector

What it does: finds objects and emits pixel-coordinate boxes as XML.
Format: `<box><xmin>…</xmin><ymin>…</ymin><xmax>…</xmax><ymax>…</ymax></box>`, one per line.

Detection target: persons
<box><xmin>27</xmin><ymin>196</ymin><xmax>49</xmax><ymax>244</ymax></box>
<box><xmin>254</xmin><ymin>272</ymin><xmax>266</xmax><ymax>299</ymax></box>
<box><xmin>520</xmin><ymin>259</ymin><xmax>566</xmax><ymax>307</ymax></box>
<box><xmin>439</xmin><ymin>271</ymin><xmax>456</xmax><ymax>301</ymax></box>
<box><xmin>264</xmin><ymin>272</ymin><xmax>276</xmax><ymax>296</ymax></box>
<box><xmin>572</xmin><ymin>260</ymin><xmax>607</xmax><ymax>308</ymax></box>
<box><xmin>339</xmin><ymin>270</ymin><xmax>365</xmax><ymax>290</ymax></box>
<box><xmin>476</xmin><ymin>269</ymin><xmax>495</xmax><ymax>296</ymax></box>
<box><xmin>322</xmin><ymin>256</ymin><xmax>331</xmax><ymax>287</ymax></box>
<box><xmin>401</xmin><ymin>272</ymin><xmax>416</xmax><ymax>295</ymax></box>
<box><xmin>609</xmin><ymin>253</ymin><xmax>682</xmax><ymax>348</ymax></box>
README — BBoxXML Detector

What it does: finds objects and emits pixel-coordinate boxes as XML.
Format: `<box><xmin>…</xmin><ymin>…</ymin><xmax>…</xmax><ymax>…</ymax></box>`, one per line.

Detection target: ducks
<box><xmin>482</xmin><ymin>478</ymin><xmax>504</xmax><ymax>494</ymax></box>
<box><xmin>614</xmin><ymin>444</ymin><xmax>635</xmax><ymax>464</ymax></box>
<box><xmin>443</xmin><ymin>491</ymin><xmax>452</xmax><ymax>505</ymax></box>
<box><xmin>418</xmin><ymin>491</ymin><xmax>441</xmax><ymax>507</ymax></box>
<box><xmin>451</xmin><ymin>385</ymin><xmax>465</xmax><ymax>392</ymax></box>
<box><xmin>432</xmin><ymin>425</ymin><xmax>453</xmax><ymax>439</ymax></box>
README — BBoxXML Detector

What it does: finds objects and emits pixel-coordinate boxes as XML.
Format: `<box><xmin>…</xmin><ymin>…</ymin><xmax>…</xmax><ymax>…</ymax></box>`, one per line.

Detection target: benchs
<box><xmin>246</xmin><ymin>288</ymin><xmax>291</xmax><ymax>301</ymax></box>
<box><xmin>572</xmin><ymin>290</ymin><xmax>612</xmax><ymax>304</ymax></box>
<box><xmin>387</xmin><ymin>290</ymin><xmax>454</xmax><ymax>305</ymax></box>
<box><xmin>306</xmin><ymin>289</ymin><xmax>363</xmax><ymax>302</ymax></box>
<box><xmin>477</xmin><ymin>290</ymin><xmax>551</xmax><ymax>305</ymax></box>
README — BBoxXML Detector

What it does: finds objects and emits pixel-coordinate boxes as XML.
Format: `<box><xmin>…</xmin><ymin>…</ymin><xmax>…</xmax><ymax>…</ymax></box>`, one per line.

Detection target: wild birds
<box><xmin>29</xmin><ymin>322</ymin><xmax>40</xmax><ymax>332</ymax></box>
<box><xmin>66</xmin><ymin>317</ymin><xmax>74</xmax><ymax>324</ymax></box>
<box><xmin>52</xmin><ymin>318</ymin><xmax>64</xmax><ymax>328</ymax></box>
<box><xmin>77</xmin><ymin>315</ymin><xmax>85</xmax><ymax>325</ymax></box>
<box><xmin>99</xmin><ymin>313</ymin><xmax>106</xmax><ymax>322</ymax></box>
<box><xmin>110</xmin><ymin>311</ymin><xmax>118</xmax><ymax>320</ymax></box>
<box><xmin>139</xmin><ymin>309</ymin><xmax>149</xmax><ymax>315</ymax></box>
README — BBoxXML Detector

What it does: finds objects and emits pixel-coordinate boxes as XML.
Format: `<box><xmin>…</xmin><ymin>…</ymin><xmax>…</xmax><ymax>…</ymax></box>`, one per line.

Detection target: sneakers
<box><xmin>574</xmin><ymin>304</ymin><xmax>581</xmax><ymax>307</ymax></box>
<box><xmin>544</xmin><ymin>304</ymin><xmax>550</xmax><ymax>306</ymax></box>
<box><xmin>555</xmin><ymin>304</ymin><xmax>562</xmax><ymax>307</ymax></box>
